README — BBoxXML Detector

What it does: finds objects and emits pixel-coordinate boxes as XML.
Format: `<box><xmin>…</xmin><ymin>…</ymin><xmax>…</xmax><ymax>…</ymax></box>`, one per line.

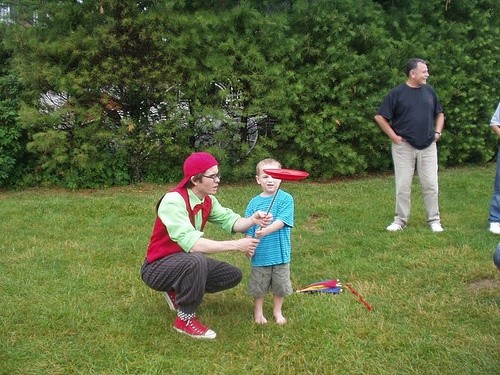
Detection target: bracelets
<box><xmin>435</xmin><ymin>131</ymin><xmax>442</xmax><ymax>136</ymax></box>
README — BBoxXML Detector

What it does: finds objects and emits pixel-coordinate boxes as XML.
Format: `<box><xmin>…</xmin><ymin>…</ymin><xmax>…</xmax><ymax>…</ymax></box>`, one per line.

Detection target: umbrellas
<box><xmin>296</xmin><ymin>278</ymin><xmax>350</xmax><ymax>294</ymax></box>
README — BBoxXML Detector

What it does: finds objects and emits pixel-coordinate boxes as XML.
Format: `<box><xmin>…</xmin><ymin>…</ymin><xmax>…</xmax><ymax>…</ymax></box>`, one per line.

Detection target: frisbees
<box><xmin>263</xmin><ymin>169</ymin><xmax>310</xmax><ymax>181</ymax></box>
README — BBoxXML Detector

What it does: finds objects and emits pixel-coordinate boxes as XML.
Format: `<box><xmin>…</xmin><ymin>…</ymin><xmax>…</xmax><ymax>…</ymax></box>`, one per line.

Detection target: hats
<box><xmin>179</xmin><ymin>151</ymin><xmax>217</xmax><ymax>188</ymax></box>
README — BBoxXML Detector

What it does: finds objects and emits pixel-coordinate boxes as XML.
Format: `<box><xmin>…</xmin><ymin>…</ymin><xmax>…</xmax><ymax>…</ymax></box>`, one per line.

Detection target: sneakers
<box><xmin>431</xmin><ymin>223</ymin><xmax>444</xmax><ymax>232</ymax></box>
<box><xmin>172</xmin><ymin>316</ymin><xmax>216</xmax><ymax>340</ymax></box>
<box><xmin>163</xmin><ymin>290</ymin><xmax>179</xmax><ymax>312</ymax></box>
<box><xmin>490</xmin><ymin>221</ymin><xmax>500</xmax><ymax>234</ymax></box>
<box><xmin>386</xmin><ymin>223</ymin><xmax>401</xmax><ymax>232</ymax></box>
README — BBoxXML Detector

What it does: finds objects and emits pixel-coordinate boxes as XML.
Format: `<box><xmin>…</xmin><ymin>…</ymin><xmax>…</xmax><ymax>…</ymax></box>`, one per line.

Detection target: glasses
<box><xmin>202</xmin><ymin>173</ymin><xmax>221</xmax><ymax>181</ymax></box>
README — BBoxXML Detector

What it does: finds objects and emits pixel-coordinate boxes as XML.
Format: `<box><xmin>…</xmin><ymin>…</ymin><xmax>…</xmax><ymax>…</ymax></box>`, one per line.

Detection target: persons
<box><xmin>140</xmin><ymin>152</ymin><xmax>274</xmax><ymax>339</ymax></box>
<box><xmin>374</xmin><ymin>58</ymin><xmax>445</xmax><ymax>232</ymax></box>
<box><xmin>241</xmin><ymin>158</ymin><xmax>294</xmax><ymax>325</ymax></box>
<box><xmin>489</xmin><ymin>103</ymin><xmax>500</xmax><ymax>235</ymax></box>
